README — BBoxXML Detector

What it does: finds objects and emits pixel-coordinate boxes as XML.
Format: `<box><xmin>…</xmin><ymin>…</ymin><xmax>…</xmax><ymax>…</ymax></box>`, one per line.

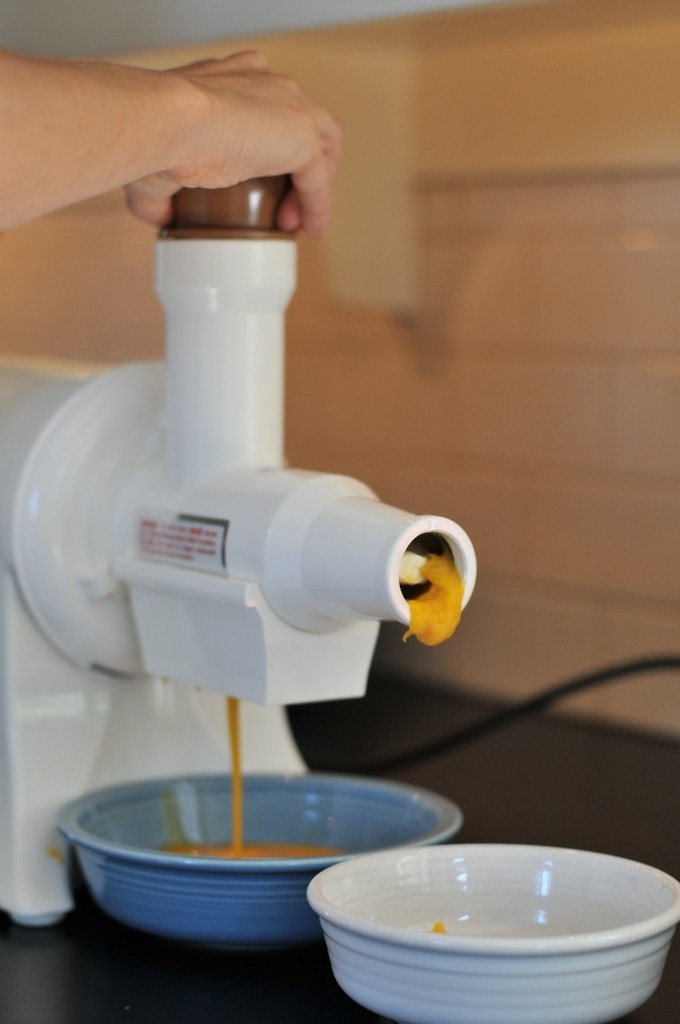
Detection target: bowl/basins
<box><xmin>306</xmin><ymin>843</ymin><xmax>680</xmax><ymax>1024</ymax></box>
<box><xmin>55</xmin><ymin>773</ymin><xmax>463</xmax><ymax>948</ymax></box>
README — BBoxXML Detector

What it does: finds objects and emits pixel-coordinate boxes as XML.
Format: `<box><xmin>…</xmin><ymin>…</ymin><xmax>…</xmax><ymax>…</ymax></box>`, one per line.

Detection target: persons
<box><xmin>0</xmin><ymin>46</ymin><xmax>341</xmax><ymax>243</ymax></box>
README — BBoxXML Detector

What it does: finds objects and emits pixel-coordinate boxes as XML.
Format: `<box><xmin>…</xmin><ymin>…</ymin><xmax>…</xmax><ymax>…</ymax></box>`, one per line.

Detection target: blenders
<box><xmin>0</xmin><ymin>174</ymin><xmax>478</xmax><ymax>925</ymax></box>
